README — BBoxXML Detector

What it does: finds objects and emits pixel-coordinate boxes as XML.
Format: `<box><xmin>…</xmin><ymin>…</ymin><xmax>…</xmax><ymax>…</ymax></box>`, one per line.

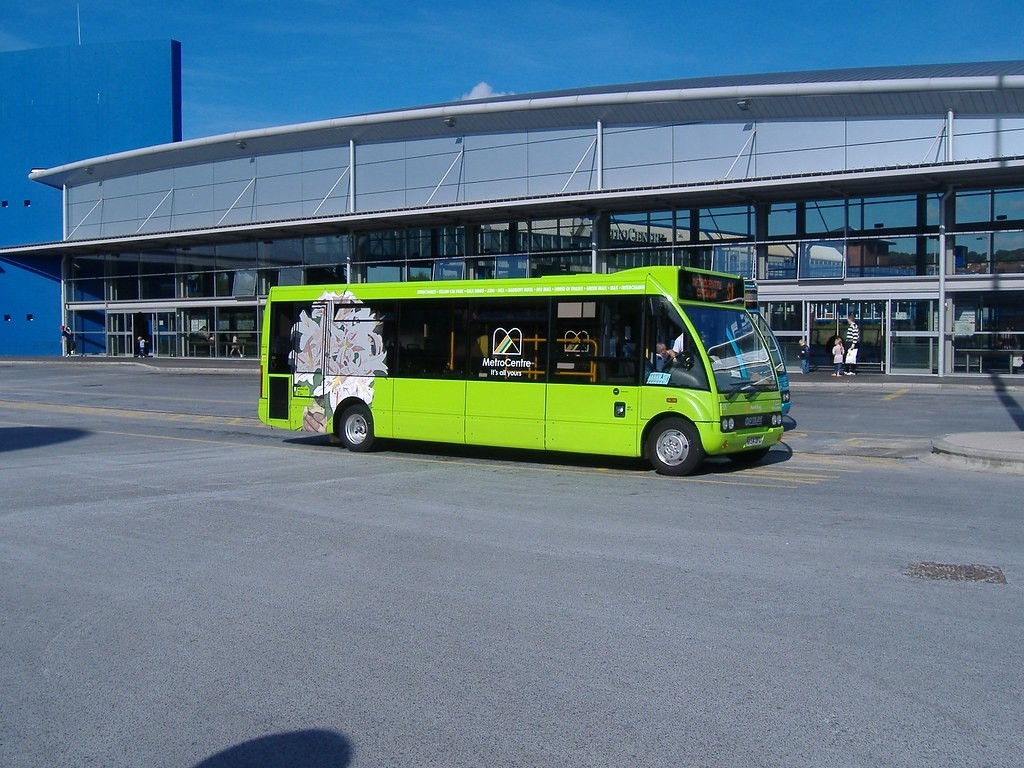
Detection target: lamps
<box><xmin>737</xmin><ymin>100</ymin><xmax>751</xmax><ymax>110</ymax></box>
<box><xmin>84</xmin><ymin>167</ymin><xmax>94</xmax><ymax>175</ymax></box>
<box><xmin>443</xmin><ymin>118</ymin><xmax>457</xmax><ymax>128</ymax></box>
<box><xmin>235</xmin><ymin>140</ymin><xmax>247</xmax><ymax>149</ymax></box>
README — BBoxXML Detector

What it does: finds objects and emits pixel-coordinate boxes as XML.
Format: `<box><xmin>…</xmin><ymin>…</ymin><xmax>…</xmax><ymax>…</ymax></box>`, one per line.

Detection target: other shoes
<box><xmin>831</xmin><ymin>374</ymin><xmax>837</xmax><ymax>377</ymax></box>
<box><xmin>847</xmin><ymin>371</ymin><xmax>856</xmax><ymax>376</ymax></box>
<box><xmin>844</xmin><ymin>371</ymin><xmax>848</xmax><ymax>375</ymax></box>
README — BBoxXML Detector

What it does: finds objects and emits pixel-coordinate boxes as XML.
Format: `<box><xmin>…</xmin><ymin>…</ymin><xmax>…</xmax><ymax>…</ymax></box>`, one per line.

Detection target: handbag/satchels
<box><xmin>845</xmin><ymin>348</ymin><xmax>858</xmax><ymax>364</ymax></box>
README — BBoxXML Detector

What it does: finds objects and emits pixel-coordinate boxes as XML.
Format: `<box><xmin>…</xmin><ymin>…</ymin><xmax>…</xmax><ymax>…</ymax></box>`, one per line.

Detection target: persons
<box><xmin>136</xmin><ymin>336</ymin><xmax>149</xmax><ymax>359</ymax></box>
<box><xmin>60</xmin><ymin>326</ymin><xmax>73</xmax><ymax>358</ymax></box>
<box><xmin>798</xmin><ymin>340</ymin><xmax>810</xmax><ymax>374</ymax></box>
<box><xmin>229</xmin><ymin>334</ymin><xmax>244</xmax><ymax>358</ymax></box>
<box><xmin>611</xmin><ymin>312</ymin><xmax>626</xmax><ymax>376</ymax></box>
<box><xmin>654</xmin><ymin>341</ymin><xmax>675</xmax><ymax>373</ymax></box>
<box><xmin>830</xmin><ymin>315</ymin><xmax>860</xmax><ymax>376</ymax></box>
<box><xmin>198</xmin><ymin>326</ymin><xmax>215</xmax><ymax>340</ymax></box>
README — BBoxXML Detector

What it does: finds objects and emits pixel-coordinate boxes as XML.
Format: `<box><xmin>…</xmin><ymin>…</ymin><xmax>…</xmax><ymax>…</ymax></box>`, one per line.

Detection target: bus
<box><xmin>258</xmin><ymin>265</ymin><xmax>784</xmax><ymax>477</ymax></box>
<box><xmin>698</xmin><ymin>282</ymin><xmax>792</xmax><ymax>419</ymax></box>
